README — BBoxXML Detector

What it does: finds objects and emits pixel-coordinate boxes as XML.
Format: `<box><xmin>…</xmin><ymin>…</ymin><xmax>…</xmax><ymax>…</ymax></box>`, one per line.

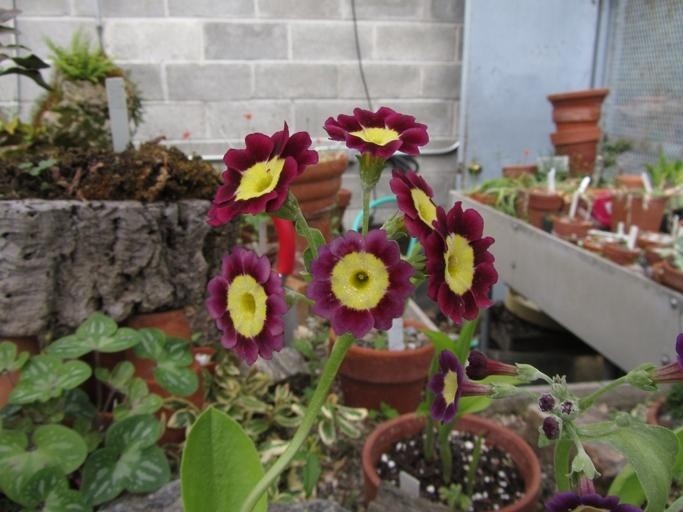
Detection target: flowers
<box><xmin>206</xmin><ymin>107</ymin><xmax>496</xmax><ymax>511</ymax></box>
<box><xmin>424</xmin><ymin>334</ymin><xmax>683</xmax><ymax>511</ymax></box>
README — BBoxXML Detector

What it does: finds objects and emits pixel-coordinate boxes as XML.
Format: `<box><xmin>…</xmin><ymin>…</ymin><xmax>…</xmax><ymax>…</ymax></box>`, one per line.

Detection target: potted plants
<box><xmin>0</xmin><ymin>309</ymin><xmax>199</xmax><ymax>512</ymax></box>
<box><xmin>467</xmin><ymin>78</ymin><xmax>682</xmax><ymax>290</ymax></box>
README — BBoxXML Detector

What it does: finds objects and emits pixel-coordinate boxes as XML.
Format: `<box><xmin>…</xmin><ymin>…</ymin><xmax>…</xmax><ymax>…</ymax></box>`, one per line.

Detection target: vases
<box><xmin>328</xmin><ymin>321</ymin><xmax>435</xmax><ymax>421</ymax></box>
<box><xmin>0</xmin><ymin>331</ymin><xmax>42</xmax><ymax>411</ymax></box>
<box><xmin>269</xmin><ymin>149</ymin><xmax>352</xmax><ymax>255</ymax></box>
<box><xmin>118</xmin><ymin>307</ymin><xmax>196</xmax><ymax>348</ymax></box>
<box><xmin>121</xmin><ymin>350</ymin><xmax>221</xmax><ymax>447</ymax></box>
<box><xmin>330</xmin><ymin>185</ymin><xmax>352</xmax><ymax>237</ymax></box>
<box><xmin>359</xmin><ymin>402</ymin><xmax>544</xmax><ymax>510</ymax></box>
